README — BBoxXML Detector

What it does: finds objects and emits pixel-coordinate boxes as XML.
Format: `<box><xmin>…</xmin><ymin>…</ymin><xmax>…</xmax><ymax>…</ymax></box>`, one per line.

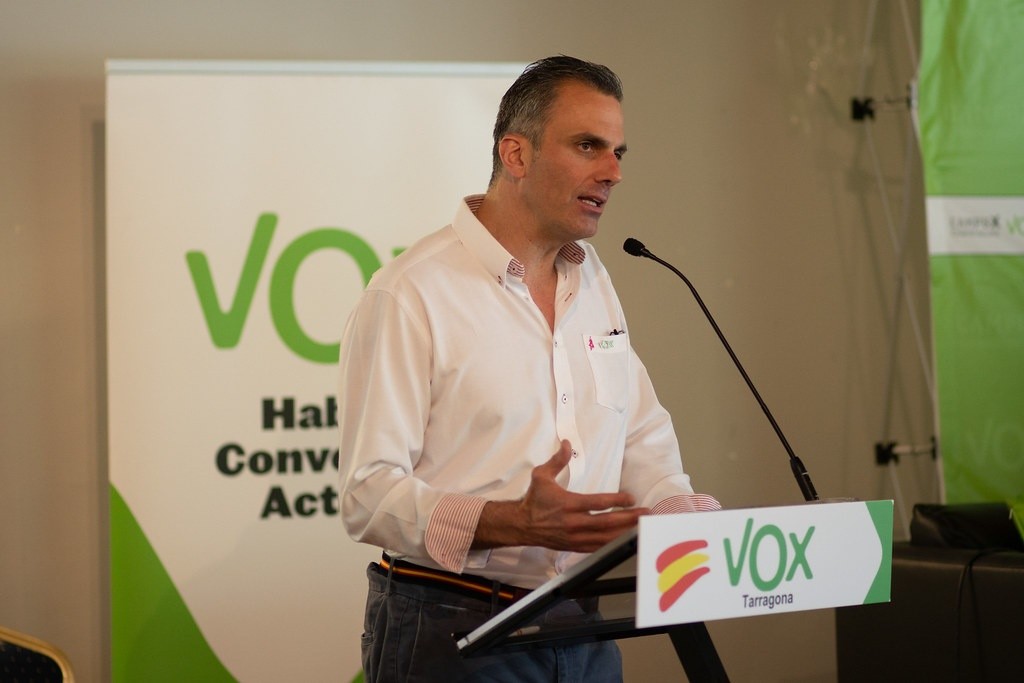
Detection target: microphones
<box><xmin>623</xmin><ymin>237</ymin><xmax>819</xmax><ymax>503</ymax></box>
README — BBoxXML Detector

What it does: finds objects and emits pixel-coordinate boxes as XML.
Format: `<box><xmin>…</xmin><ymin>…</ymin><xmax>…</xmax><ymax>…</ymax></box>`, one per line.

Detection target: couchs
<box><xmin>836</xmin><ymin>501</ymin><xmax>1024</xmax><ymax>683</ymax></box>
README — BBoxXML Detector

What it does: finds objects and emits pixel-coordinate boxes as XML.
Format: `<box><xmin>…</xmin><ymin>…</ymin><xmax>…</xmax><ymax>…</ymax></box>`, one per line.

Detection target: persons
<box><xmin>337</xmin><ymin>53</ymin><xmax>722</xmax><ymax>683</ymax></box>
